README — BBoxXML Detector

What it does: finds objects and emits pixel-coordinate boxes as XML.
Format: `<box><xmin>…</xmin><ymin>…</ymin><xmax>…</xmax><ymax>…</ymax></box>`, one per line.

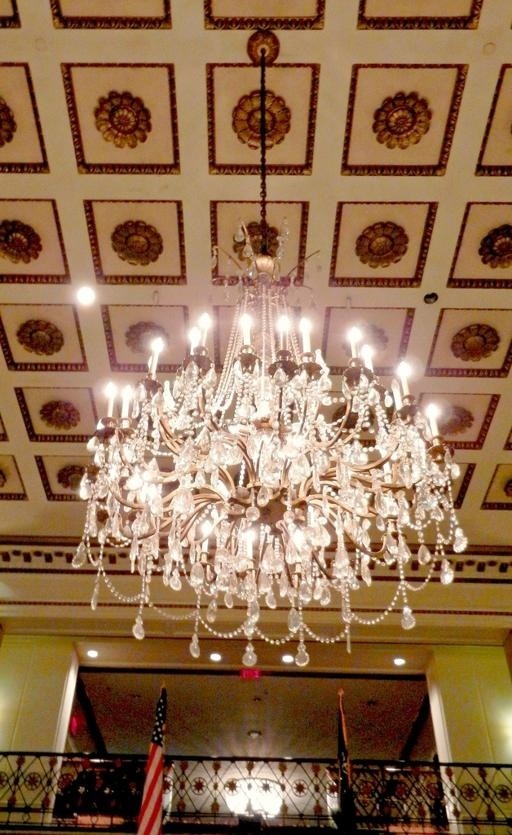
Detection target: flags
<box><xmin>136</xmin><ymin>683</ymin><xmax>168</xmax><ymax>835</ymax></box>
<box><xmin>335</xmin><ymin>695</ymin><xmax>356</xmax><ymax>831</ymax></box>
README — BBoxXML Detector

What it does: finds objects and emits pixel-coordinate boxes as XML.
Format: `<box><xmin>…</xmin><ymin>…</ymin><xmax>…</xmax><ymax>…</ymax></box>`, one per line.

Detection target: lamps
<box><xmin>71</xmin><ymin>47</ymin><xmax>467</xmax><ymax>669</ymax></box>
